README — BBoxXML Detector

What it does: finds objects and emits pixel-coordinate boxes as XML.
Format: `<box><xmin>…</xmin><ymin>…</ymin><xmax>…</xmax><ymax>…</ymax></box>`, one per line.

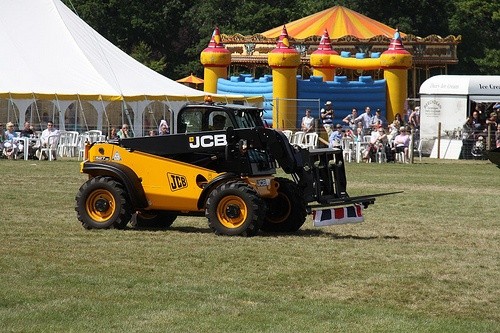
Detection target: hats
<box><xmin>376</xmin><ymin>109</ymin><xmax>382</xmax><ymax>112</ymax></box>
<box><xmin>335</xmin><ymin>124</ymin><xmax>343</xmax><ymax>128</ymax></box>
<box><xmin>399</xmin><ymin>126</ymin><xmax>407</xmax><ymax>131</ymax></box>
<box><xmin>324</xmin><ymin>101</ymin><xmax>332</xmax><ymax>105</ymax></box>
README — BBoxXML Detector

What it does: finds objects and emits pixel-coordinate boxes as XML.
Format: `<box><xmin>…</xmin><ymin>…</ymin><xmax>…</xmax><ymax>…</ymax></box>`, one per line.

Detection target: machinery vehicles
<box><xmin>74</xmin><ymin>96</ymin><xmax>404</xmax><ymax>238</ymax></box>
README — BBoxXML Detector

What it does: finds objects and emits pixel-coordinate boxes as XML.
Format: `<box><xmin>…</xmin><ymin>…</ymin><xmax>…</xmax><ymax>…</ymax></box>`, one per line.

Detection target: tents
<box><xmin>0</xmin><ymin>0</ymin><xmax>265</xmax><ymax>136</ymax></box>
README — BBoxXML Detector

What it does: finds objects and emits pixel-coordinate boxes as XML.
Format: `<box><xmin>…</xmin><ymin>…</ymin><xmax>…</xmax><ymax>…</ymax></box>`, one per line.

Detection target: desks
<box><xmin>309</xmin><ymin>148</ymin><xmax>341</xmax><ymax>164</ymax></box>
<box><xmin>12</xmin><ymin>137</ymin><xmax>40</xmax><ymax>160</ymax></box>
<box><xmin>349</xmin><ymin>142</ymin><xmax>371</xmax><ymax>164</ymax></box>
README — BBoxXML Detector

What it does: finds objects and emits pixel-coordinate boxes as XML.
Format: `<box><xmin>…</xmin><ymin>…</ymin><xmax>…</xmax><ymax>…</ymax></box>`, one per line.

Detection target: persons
<box><xmin>301</xmin><ymin>104</ymin><xmax>500</xmax><ymax>164</ymax></box>
<box><xmin>0</xmin><ymin>119</ymin><xmax>170</xmax><ymax>161</ymax></box>
<box><xmin>212</xmin><ymin>115</ymin><xmax>233</xmax><ymax>131</ymax></box>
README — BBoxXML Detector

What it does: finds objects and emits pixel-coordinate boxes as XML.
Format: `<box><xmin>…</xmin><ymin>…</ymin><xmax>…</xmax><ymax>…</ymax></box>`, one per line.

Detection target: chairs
<box><xmin>0</xmin><ymin>130</ymin><xmax>106</xmax><ymax>161</ymax></box>
<box><xmin>282</xmin><ymin>130</ymin><xmax>422</xmax><ymax>164</ymax></box>
